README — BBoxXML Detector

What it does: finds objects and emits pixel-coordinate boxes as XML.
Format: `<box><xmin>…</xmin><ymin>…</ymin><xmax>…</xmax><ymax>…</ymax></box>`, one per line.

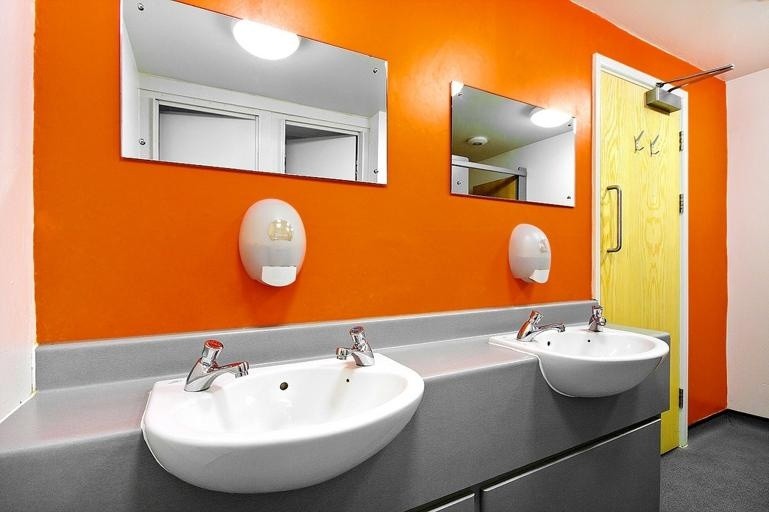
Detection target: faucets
<box><xmin>518</xmin><ymin>310</ymin><xmax>565</xmax><ymax>340</ymax></box>
<box><xmin>337</xmin><ymin>325</ymin><xmax>374</xmax><ymax>368</ymax></box>
<box><xmin>591</xmin><ymin>308</ymin><xmax>606</xmax><ymax>333</ymax></box>
<box><xmin>185</xmin><ymin>339</ymin><xmax>248</xmax><ymax>391</ymax></box>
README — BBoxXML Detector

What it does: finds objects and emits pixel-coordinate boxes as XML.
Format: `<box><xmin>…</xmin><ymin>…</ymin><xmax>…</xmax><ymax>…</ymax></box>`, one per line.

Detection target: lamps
<box><xmin>233</xmin><ymin>19</ymin><xmax>300</xmax><ymax>60</ymax></box>
<box><xmin>529</xmin><ymin>106</ymin><xmax>571</xmax><ymax>128</ymax></box>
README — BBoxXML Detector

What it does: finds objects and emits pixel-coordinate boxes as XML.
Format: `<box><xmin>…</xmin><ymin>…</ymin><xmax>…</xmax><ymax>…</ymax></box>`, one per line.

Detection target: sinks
<box><xmin>143</xmin><ymin>353</ymin><xmax>425</xmax><ymax>494</ymax></box>
<box><xmin>488</xmin><ymin>323</ymin><xmax>672</xmax><ymax>401</ymax></box>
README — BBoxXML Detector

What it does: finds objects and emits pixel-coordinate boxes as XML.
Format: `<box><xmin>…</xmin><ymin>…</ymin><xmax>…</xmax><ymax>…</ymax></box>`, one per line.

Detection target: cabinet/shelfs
<box><xmin>426</xmin><ymin>418</ymin><xmax>661</xmax><ymax>512</ymax></box>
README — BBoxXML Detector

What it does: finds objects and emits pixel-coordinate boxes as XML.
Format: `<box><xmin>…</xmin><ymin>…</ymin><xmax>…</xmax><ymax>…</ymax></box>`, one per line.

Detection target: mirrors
<box><xmin>120</xmin><ymin>0</ymin><xmax>388</xmax><ymax>185</ymax></box>
<box><xmin>450</xmin><ymin>81</ymin><xmax>576</xmax><ymax>208</ymax></box>
